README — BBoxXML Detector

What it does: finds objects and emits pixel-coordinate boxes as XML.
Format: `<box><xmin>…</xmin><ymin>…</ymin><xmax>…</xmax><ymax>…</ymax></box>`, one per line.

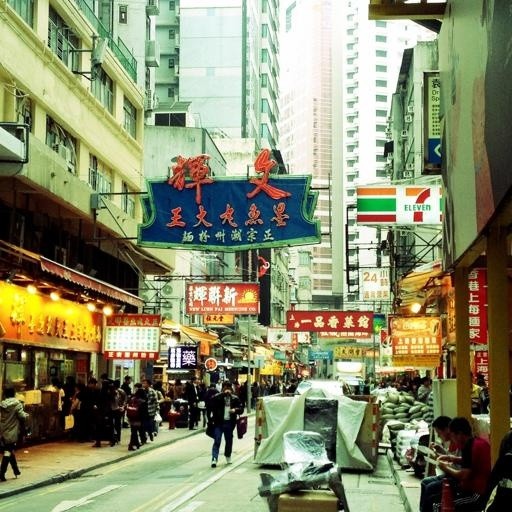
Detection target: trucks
<box><xmin>333</xmin><ymin>360</ymin><xmax>367</xmax><ymax>395</ymax></box>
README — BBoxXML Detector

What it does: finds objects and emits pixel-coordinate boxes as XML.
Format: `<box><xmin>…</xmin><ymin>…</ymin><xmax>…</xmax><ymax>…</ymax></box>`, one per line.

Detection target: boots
<box><xmin>9</xmin><ymin>455</ymin><xmax>21</xmax><ymax>479</ymax></box>
<box><xmin>0</xmin><ymin>456</ymin><xmax>10</xmax><ymax>481</ymax></box>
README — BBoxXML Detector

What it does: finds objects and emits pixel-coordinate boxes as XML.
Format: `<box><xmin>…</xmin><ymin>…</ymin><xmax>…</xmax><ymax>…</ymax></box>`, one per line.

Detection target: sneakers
<box><xmin>211</xmin><ymin>461</ymin><xmax>216</xmax><ymax>468</ymax></box>
<box><xmin>226</xmin><ymin>456</ymin><xmax>232</xmax><ymax>464</ymax></box>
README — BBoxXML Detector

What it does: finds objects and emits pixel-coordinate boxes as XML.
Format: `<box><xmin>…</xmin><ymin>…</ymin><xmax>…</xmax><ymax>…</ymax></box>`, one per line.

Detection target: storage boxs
<box><xmin>277</xmin><ymin>491</ymin><xmax>340</xmax><ymax>512</ymax></box>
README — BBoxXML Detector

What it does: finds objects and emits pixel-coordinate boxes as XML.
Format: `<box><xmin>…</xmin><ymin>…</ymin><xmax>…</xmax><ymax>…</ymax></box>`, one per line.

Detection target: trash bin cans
<box><xmin>173</xmin><ymin>399</ymin><xmax>189</xmax><ymax>428</ymax></box>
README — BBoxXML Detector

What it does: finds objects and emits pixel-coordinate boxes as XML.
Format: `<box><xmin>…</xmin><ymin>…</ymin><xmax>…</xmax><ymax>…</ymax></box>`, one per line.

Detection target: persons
<box><xmin>0</xmin><ymin>388</ymin><xmax>29</xmax><ymax>481</ymax></box>
<box><xmin>46</xmin><ymin>374</ymin><xmax>296</xmax><ymax>468</ymax></box>
<box><xmin>379</xmin><ymin>374</ymin><xmax>512</xmax><ymax>512</ymax></box>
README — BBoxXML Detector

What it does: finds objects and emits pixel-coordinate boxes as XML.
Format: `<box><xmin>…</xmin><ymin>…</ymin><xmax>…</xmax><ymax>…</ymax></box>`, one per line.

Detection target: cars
<box><xmin>294</xmin><ymin>378</ymin><xmax>351</xmax><ymax>397</ymax></box>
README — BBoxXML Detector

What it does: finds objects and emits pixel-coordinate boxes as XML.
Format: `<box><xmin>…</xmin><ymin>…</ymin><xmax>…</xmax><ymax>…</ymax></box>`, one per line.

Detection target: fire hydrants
<box><xmin>167</xmin><ymin>408</ymin><xmax>181</xmax><ymax>430</ymax></box>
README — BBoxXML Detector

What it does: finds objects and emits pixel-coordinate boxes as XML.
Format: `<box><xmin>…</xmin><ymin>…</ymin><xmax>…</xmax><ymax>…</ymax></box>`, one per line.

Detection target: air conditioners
<box><xmin>172</xmin><ymin>4</ymin><xmax>181</xmax><ymax>103</ymax></box>
<box><xmin>148</xmin><ymin>0</ymin><xmax>159</xmax><ymax>9</ymax></box>
<box><xmin>144</xmin><ymin>39</ymin><xmax>161</xmax><ymax>67</ymax></box>
<box><xmin>51</xmin><ymin>143</ymin><xmax>71</xmax><ymax>163</ymax></box>
<box><xmin>377</xmin><ymin>103</ymin><xmax>422</xmax><ymax>183</ymax></box>
<box><xmin>144</xmin><ymin>87</ymin><xmax>158</xmax><ymax>112</ymax></box>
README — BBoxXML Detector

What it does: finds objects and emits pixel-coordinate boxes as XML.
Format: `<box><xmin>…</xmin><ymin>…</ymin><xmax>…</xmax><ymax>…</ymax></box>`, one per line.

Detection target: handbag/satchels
<box><xmin>206</xmin><ymin>416</ymin><xmax>215</xmax><ymax>439</ymax></box>
<box><xmin>237</xmin><ymin>416</ymin><xmax>248</xmax><ymax>439</ymax></box>
